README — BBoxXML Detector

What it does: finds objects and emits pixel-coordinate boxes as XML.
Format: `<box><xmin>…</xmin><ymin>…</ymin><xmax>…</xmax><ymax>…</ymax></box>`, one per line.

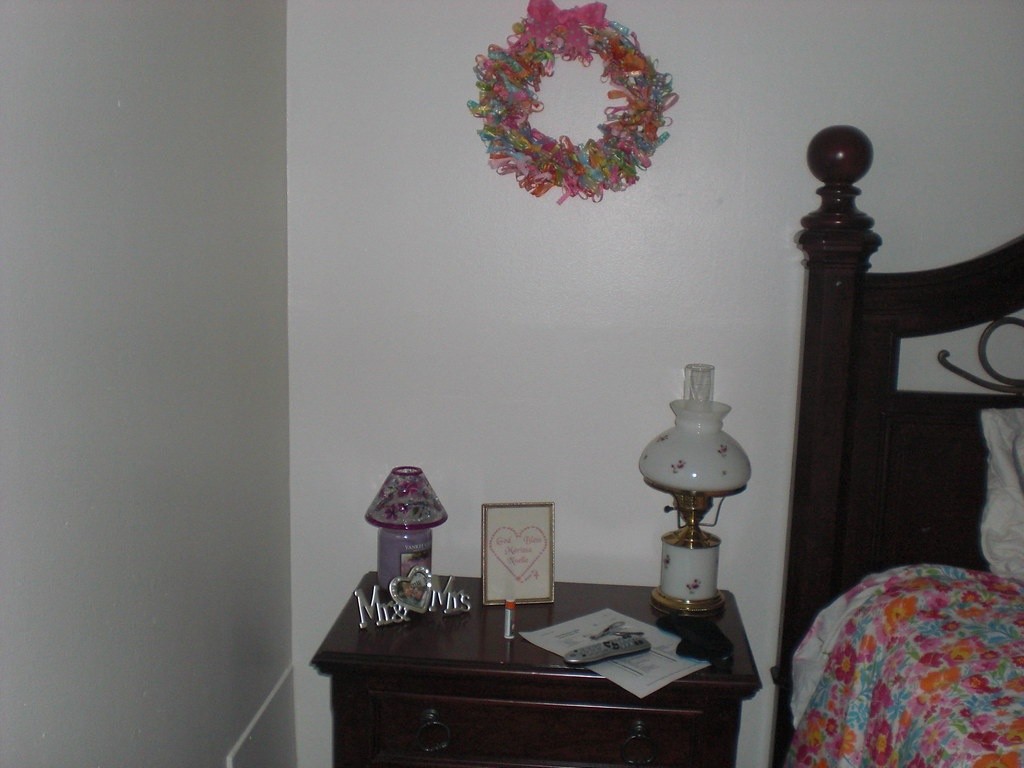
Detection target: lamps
<box><xmin>638</xmin><ymin>363</ymin><xmax>751</xmax><ymax>618</ymax></box>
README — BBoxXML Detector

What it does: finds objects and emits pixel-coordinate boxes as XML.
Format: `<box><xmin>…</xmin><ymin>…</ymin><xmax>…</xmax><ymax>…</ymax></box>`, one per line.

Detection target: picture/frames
<box><xmin>481</xmin><ymin>503</ymin><xmax>557</xmax><ymax>603</ymax></box>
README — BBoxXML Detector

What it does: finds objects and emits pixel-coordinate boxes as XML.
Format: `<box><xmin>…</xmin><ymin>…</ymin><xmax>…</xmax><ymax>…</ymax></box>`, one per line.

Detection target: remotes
<box><xmin>563</xmin><ymin>636</ymin><xmax>651</xmax><ymax>667</ymax></box>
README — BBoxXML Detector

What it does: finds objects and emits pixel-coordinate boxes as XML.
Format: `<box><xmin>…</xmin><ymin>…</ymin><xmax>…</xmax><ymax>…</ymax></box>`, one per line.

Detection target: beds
<box><xmin>769</xmin><ymin>125</ymin><xmax>1024</xmax><ymax>768</ymax></box>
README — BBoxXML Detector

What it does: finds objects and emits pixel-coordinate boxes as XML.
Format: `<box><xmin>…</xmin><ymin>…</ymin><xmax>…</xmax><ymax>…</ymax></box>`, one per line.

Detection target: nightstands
<box><xmin>310</xmin><ymin>571</ymin><xmax>764</xmax><ymax>768</ymax></box>
